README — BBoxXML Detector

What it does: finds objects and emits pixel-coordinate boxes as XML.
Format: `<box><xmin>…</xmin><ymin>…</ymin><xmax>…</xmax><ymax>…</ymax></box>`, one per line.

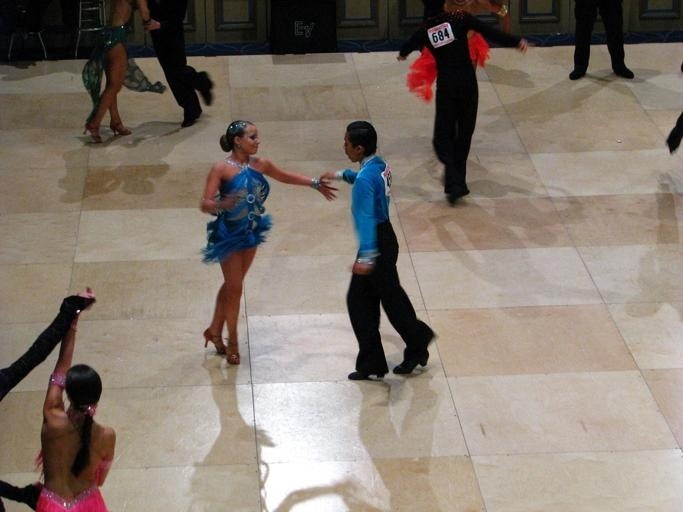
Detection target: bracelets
<box><xmin>142</xmin><ymin>18</ymin><xmax>151</xmax><ymax>24</ymax></box>
<box><xmin>311</xmin><ymin>178</ymin><xmax>319</xmax><ymax>190</ymax></box>
<box><xmin>215</xmin><ymin>202</ymin><xmax>222</xmax><ymax>216</ymax></box>
<box><xmin>71</xmin><ymin>320</ymin><xmax>77</xmax><ymax>330</ymax></box>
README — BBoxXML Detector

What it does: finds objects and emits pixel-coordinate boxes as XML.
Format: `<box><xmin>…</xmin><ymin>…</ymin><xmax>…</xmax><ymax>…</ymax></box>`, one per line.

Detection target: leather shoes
<box><xmin>348</xmin><ymin>371</ymin><xmax>385</xmax><ymax>380</ymax></box>
<box><xmin>181</xmin><ymin>110</ymin><xmax>202</xmax><ymax>127</ymax></box>
<box><xmin>569</xmin><ymin>71</ymin><xmax>585</xmax><ymax>80</ymax></box>
<box><xmin>201</xmin><ymin>78</ymin><xmax>213</xmax><ymax>106</ymax></box>
<box><xmin>613</xmin><ymin>68</ymin><xmax>634</xmax><ymax>79</ymax></box>
<box><xmin>393</xmin><ymin>350</ymin><xmax>429</xmax><ymax>373</ymax></box>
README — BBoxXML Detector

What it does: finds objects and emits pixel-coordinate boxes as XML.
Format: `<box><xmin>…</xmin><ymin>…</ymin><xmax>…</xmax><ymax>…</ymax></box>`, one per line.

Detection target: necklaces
<box><xmin>225</xmin><ymin>156</ymin><xmax>253</xmax><ymax>168</ymax></box>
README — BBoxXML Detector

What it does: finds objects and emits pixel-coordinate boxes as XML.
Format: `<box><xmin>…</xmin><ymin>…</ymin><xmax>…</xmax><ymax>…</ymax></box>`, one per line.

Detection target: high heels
<box><xmin>203</xmin><ymin>328</ymin><xmax>227</xmax><ymax>358</ymax></box>
<box><xmin>83</xmin><ymin>119</ymin><xmax>103</xmax><ymax>143</ymax></box>
<box><xmin>110</xmin><ymin>119</ymin><xmax>133</xmax><ymax>136</ymax></box>
<box><xmin>223</xmin><ymin>335</ymin><xmax>240</xmax><ymax>364</ymax></box>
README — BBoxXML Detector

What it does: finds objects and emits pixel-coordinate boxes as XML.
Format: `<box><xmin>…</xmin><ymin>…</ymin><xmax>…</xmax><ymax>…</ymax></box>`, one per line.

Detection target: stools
<box><xmin>3</xmin><ymin>0</ymin><xmax>47</xmax><ymax>61</ymax></box>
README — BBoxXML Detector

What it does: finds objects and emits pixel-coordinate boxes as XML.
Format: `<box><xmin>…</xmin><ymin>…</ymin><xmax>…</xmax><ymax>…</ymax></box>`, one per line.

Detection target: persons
<box><xmin>36</xmin><ymin>286</ymin><xmax>116</xmax><ymax>512</ymax></box>
<box><xmin>200</xmin><ymin>120</ymin><xmax>339</xmax><ymax>364</ymax></box>
<box><xmin>80</xmin><ymin>0</ymin><xmax>151</xmax><ymax>141</ymax></box>
<box><xmin>665</xmin><ymin>60</ymin><xmax>683</xmax><ymax>154</ymax></box>
<box><xmin>407</xmin><ymin>0</ymin><xmax>513</xmax><ymax>103</ymax></box>
<box><xmin>145</xmin><ymin>0</ymin><xmax>214</xmax><ymax>129</ymax></box>
<box><xmin>319</xmin><ymin>120</ymin><xmax>433</xmax><ymax>378</ymax></box>
<box><xmin>396</xmin><ymin>0</ymin><xmax>529</xmax><ymax>204</ymax></box>
<box><xmin>568</xmin><ymin>0</ymin><xmax>635</xmax><ymax>81</ymax></box>
<box><xmin>0</xmin><ymin>293</ymin><xmax>98</xmax><ymax>512</ymax></box>
